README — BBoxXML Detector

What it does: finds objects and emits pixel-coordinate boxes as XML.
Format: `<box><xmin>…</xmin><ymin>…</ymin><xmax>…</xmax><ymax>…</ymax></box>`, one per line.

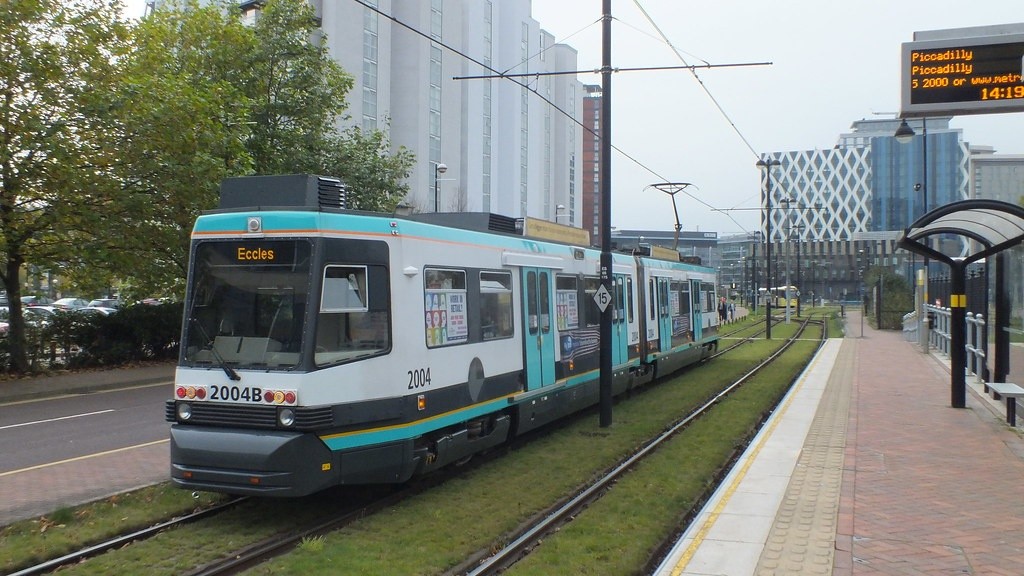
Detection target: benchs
<box><xmin>984</xmin><ymin>382</ymin><xmax>1024</xmax><ymax>428</ymax></box>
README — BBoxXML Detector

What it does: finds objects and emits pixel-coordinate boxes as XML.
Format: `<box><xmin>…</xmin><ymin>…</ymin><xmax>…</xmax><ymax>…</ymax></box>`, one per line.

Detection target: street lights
<box><xmin>892</xmin><ymin>116</ymin><xmax>929</xmax><ymax>355</ymax></box>
<box><xmin>755</xmin><ymin>158</ymin><xmax>781</xmax><ymax>341</ymax></box>
<box><xmin>779</xmin><ymin>197</ymin><xmax>796</xmax><ymax>324</ymax></box>
<box><xmin>792</xmin><ymin>225</ymin><xmax>805</xmax><ymax>317</ymax></box>
<box><xmin>555</xmin><ymin>203</ymin><xmax>566</xmax><ymax>224</ymax></box>
<box><xmin>434</xmin><ymin>162</ymin><xmax>449</xmax><ymax>212</ymax></box>
<box><xmin>718</xmin><ymin>256</ymin><xmax>749</xmax><ymax>307</ymax></box>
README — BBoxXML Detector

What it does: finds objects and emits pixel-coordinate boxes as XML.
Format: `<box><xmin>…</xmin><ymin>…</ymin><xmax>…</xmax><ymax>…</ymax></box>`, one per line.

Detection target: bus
<box><xmin>164</xmin><ymin>175</ymin><xmax>720</xmax><ymax>500</ymax></box>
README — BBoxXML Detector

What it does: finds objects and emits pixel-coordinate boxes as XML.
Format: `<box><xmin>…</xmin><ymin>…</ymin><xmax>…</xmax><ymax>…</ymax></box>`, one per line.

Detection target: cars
<box><xmin>0</xmin><ymin>292</ymin><xmax>178</xmax><ymax>340</ymax></box>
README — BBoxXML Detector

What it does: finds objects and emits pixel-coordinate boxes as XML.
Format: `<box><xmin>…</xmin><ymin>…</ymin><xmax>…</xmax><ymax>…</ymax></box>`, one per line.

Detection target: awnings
<box><xmin>894</xmin><ymin>198</ymin><xmax>1024</xmax><ymax>408</ymax></box>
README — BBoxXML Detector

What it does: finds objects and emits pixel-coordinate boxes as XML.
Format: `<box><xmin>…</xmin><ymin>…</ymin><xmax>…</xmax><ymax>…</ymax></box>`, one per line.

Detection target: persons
<box><xmin>717</xmin><ymin>296</ymin><xmax>736</xmax><ymax>321</ymax></box>
